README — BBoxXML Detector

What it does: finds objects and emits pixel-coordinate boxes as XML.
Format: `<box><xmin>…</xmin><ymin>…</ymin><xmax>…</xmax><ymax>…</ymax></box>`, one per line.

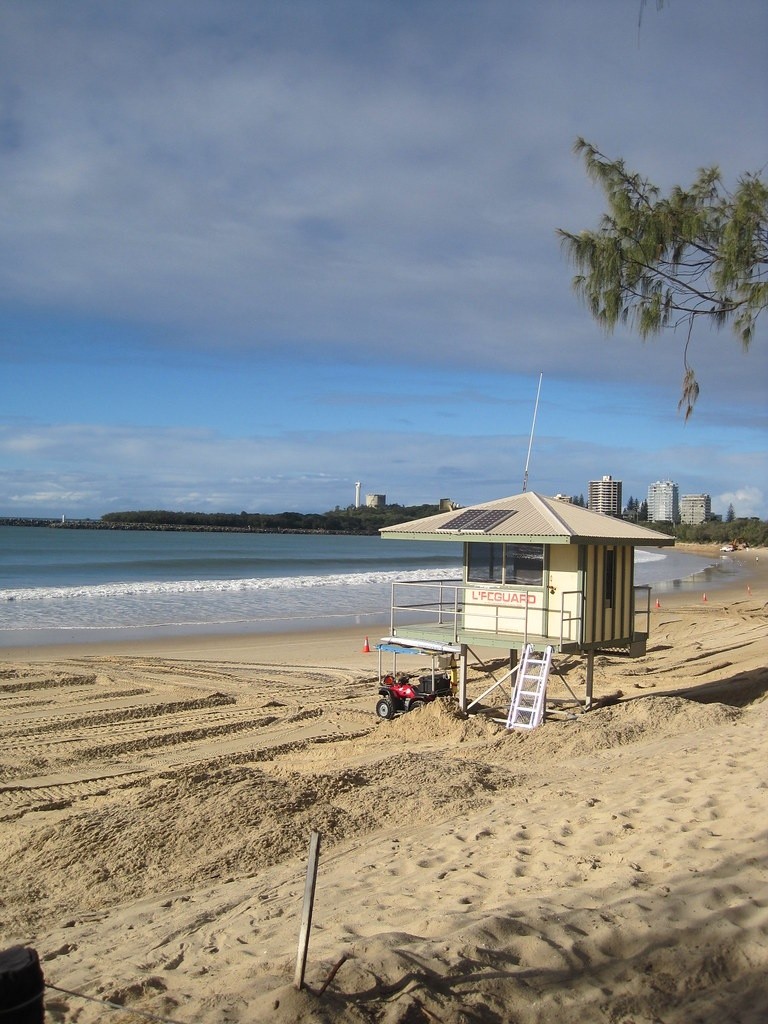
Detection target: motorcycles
<box><xmin>376</xmin><ymin>671</ymin><xmax>453</xmax><ymax>719</ymax></box>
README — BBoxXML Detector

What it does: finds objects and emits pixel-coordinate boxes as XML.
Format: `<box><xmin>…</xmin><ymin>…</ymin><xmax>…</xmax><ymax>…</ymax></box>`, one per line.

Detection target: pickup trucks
<box><xmin>719</xmin><ymin>546</ymin><xmax>734</xmax><ymax>552</ymax></box>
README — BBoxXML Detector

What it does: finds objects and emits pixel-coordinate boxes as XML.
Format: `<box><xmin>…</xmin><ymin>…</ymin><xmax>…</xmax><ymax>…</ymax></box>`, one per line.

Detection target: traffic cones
<box><xmin>655</xmin><ymin>598</ymin><xmax>660</xmax><ymax>608</ymax></box>
<box><xmin>361</xmin><ymin>636</ymin><xmax>370</xmax><ymax>653</ymax></box>
<box><xmin>703</xmin><ymin>592</ymin><xmax>707</xmax><ymax>602</ymax></box>
<box><xmin>747</xmin><ymin>584</ymin><xmax>752</xmax><ymax>596</ymax></box>
